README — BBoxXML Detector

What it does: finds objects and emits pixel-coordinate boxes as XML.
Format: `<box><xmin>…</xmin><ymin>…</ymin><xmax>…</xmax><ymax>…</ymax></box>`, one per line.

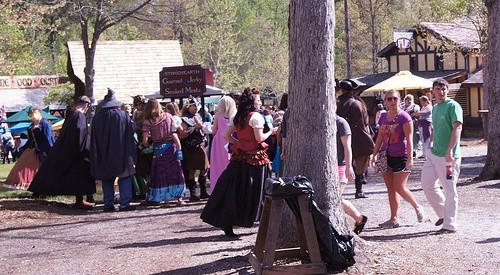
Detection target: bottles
<box><xmin>446</xmin><ymin>160</ymin><xmax>452</xmax><ymax>179</ymax></box>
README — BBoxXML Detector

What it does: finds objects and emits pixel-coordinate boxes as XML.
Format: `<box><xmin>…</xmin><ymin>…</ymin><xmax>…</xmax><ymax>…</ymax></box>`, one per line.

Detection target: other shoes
<box><xmin>119</xmin><ymin>205</ymin><xmax>137</xmax><ymax>211</ymax></box>
<box><xmin>378</xmin><ymin>220</ymin><xmax>399</xmax><ymax>228</ymax></box>
<box><xmin>76</xmin><ymin>200</ymin><xmax>95</xmax><ymax>209</ymax></box>
<box><xmin>416</xmin><ymin>205</ymin><xmax>423</xmax><ymax>223</ymax></box>
<box><xmin>436</xmin><ymin>228</ymin><xmax>456</xmax><ymax>234</ymax></box>
<box><xmin>355</xmin><ymin>193</ymin><xmax>367</xmax><ymax>198</ymax></box>
<box><xmin>435</xmin><ymin>218</ymin><xmax>443</xmax><ymax>226</ymax></box>
<box><xmin>103</xmin><ymin>205</ymin><xmax>115</xmax><ymax>211</ymax></box>
<box><xmin>354</xmin><ymin>215</ymin><xmax>368</xmax><ymax>235</ymax></box>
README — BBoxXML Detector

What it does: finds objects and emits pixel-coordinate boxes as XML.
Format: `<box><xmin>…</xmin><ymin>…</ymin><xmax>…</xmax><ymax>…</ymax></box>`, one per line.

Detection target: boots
<box><xmin>188</xmin><ymin>178</ymin><xmax>200</xmax><ymax>201</ymax></box>
<box><xmin>198</xmin><ymin>175</ymin><xmax>210</xmax><ymax>199</ymax></box>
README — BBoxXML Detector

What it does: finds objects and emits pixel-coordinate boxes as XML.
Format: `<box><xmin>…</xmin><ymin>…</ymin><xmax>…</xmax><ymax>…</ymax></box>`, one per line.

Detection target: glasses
<box><xmin>386</xmin><ymin>97</ymin><xmax>399</xmax><ymax>101</ymax></box>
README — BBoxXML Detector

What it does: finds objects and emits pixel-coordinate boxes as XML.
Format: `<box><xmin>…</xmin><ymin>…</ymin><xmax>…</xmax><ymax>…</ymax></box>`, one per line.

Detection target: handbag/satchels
<box><xmin>374</xmin><ymin>150</ymin><xmax>390</xmax><ymax>175</ymax></box>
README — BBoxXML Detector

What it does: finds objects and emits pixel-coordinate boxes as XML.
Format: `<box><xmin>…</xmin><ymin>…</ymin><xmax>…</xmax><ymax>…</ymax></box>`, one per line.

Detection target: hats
<box><xmin>98</xmin><ymin>87</ymin><xmax>124</xmax><ymax>108</ymax></box>
<box><xmin>340</xmin><ymin>79</ymin><xmax>359</xmax><ymax>91</ymax></box>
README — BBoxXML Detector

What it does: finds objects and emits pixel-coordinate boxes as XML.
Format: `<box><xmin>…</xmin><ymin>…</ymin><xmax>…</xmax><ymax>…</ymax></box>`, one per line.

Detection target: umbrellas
<box><xmin>0</xmin><ymin>105</ymin><xmax>67</xmax><ymax>132</ymax></box>
<box><xmin>145</xmin><ymin>81</ymin><xmax>224</xmax><ymax>100</ymax></box>
<box><xmin>360</xmin><ymin>70</ymin><xmax>435</xmax><ymax>97</ymax></box>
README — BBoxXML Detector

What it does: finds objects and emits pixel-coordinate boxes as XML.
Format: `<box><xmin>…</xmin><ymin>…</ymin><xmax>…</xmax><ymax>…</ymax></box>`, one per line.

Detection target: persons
<box><xmin>123</xmin><ymin>87</ymin><xmax>290</xmax><ymax>201</ymax></box>
<box><xmin>335</xmin><ymin>115</ymin><xmax>367</xmax><ymax>235</ymax></box>
<box><xmin>4</xmin><ymin>106</ymin><xmax>55</xmax><ymax>190</ymax></box>
<box><xmin>421</xmin><ymin>78</ymin><xmax>464</xmax><ymax>235</ymax></box>
<box><xmin>201</xmin><ymin>87</ymin><xmax>281</xmax><ymax>240</ymax></box>
<box><xmin>371</xmin><ymin>89</ymin><xmax>425</xmax><ymax>229</ymax></box>
<box><xmin>0</xmin><ymin>126</ymin><xmax>29</xmax><ymax>162</ymax></box>
<box><xmin>89</xmin><ymin>88</ymin><xmax>139</xmax><ymax>212</ymax></box>
<box><xmin>142</xmin><ymin>97</ymin><xmax>188</xmax><ymax>207</ymax></box>
<box><xmin>0</xmin><ymin>127</ymin><xmax>28</xmax><ymax>164</ymax></box>
<box><xmin>374</xmin><ymin>94</ymin><xmax>433</xmax><ymax>160</ymax></box>
<box><xmin>27</xmin><ymin>95</ymin><xmax>97</xmax><ymax>210</ymax></box>
<box><xmin>336</xmin><ymin>78</ymin><xmax>372</xmax><ymax>198</ymax></box>
